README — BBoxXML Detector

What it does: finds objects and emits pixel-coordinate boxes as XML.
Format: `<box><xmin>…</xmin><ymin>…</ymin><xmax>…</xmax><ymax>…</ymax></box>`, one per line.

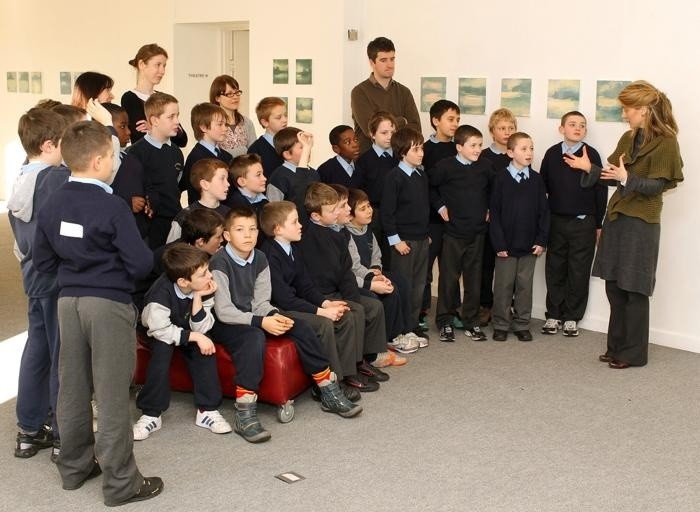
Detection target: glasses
<box><xmin>220</xmin><ymin>90</ymin><xmax>244</xmax><ymax>98</ymax></box>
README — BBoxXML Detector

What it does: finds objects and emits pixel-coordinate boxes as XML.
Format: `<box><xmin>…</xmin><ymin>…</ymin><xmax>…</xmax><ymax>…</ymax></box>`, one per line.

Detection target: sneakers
<box><xmin>345</xmin><ymin>360</ymin><xmax>390</xmax><ymax>392</ymax></box>
<box><xmin>371</xmin><ymin>349</ymin><xmax>407</xmax><ymax>367</ymax></box>
<box><xmin>418</xmin><ymin>311</ymin><xmax>430</xmax><ymax>332</ymax></box>
<box><xmin>493</xmin><ymin>329</ymin><xmax>507</xmax><ymax>342</ymax></box>
<box><xmin>464</xmin><ymin>326</ymin><xmax>486</xmax><ymax>341</ymax></box>
<box><xmin>514</xmin><ymin>329</ymin><xmax>533</xmax><ymax>341</ymax></box>
<box><xmin>312</xmin><ymin>382</ymin><xmax>364</xmax><ymax>419</ymax></box>
<box><xmin>478</xmin><ymin>304</ymin><xmax>491</xmax><ymax>327</ymax></box>
<box><xmin>105</xmin><ymin>476</ymin><xmax>163</xmax><ymax>507</ymax></box>
<box><xmin>389</xmin><ymin>332</ymin><xmax>430</xmax><ymax>354</ymax></box>
<box><xmin>434</xmin><ymin>311</ymin><xmax>465</xmax><ymax>328</ymax></box>
<box><xmin>439</xmin><ymin>323</ymin><xmax>456</xmax><ymax>342</ymax></box>
<box><xmin>194</xmin><ymin>408</ymin><xmax>231</xmax><ymax>433</ymax></box>
<box><xmin>63</xmin><ymin>456</ymin><xmax>103</xmax><ymax>490</ymax></box>
<box><xmin>232</xmin><ymin>401</ymin><xmax>270</xmax><ymax>443</ymax></box>
<box><xmin>14</xmin><ymin>398</ymin><xmax>99</xmax><ymax>463</ymax></box>
<box><xmin>131</xmin><ymin>412</ymin><xmax>162</xmax><ymax>440</ymax></box>
<box><xmin>540</xmin><ymin>318</ymin><xmax>561</xmax><ymax>336</ymax></box>
<box><xmin>563</xmin><ymin>319</ymin><xmax>579</xmax><ymax>337</ymax></box>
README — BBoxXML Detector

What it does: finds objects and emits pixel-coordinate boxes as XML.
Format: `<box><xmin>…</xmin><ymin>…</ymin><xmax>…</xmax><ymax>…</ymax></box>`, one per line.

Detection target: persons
<box><xmin>351</xmin><ymin>38</ymin><xmax>422</xmax><ymax>158</ymax></box>
<box><xmin>539</xmin><ymin>110</ymin><xmax>608</xmax><ymax>337</ymax></box>
<box><xmin>379</xmin><ymin>98</ymin><xmax>550</xmax><ymax>355</ymax></box>
<box><xmin>7</xmin><ymin>36</ymin><xmax>407</xmax><ymax>508</ymax></box>
<box><xmin>562</xmin><ymin>81</ymin><xmax>684</xmax><ymax>369</ymax></box>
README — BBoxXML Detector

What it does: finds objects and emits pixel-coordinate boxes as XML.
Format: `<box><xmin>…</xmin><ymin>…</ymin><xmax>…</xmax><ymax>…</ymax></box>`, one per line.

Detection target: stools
<box><xmin>130</xmin><ymin>336</ymin><xmax>312</xmax><ymax>423</ymax></box>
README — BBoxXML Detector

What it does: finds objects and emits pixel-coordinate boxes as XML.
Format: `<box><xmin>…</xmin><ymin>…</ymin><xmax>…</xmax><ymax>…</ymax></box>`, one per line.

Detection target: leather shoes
<box><xmin>600</xmin><ymin>353</ymin><xmax>632</xmax><ymax>370</ymax></box>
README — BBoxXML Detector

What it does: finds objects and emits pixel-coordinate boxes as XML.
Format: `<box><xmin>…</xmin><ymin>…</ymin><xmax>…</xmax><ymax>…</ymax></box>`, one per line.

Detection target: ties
<box><xmin>383</xmin><ymin>151</ymin><xmax>392</xmax><ymax>158</ymax></box>
<box><xmin>517</xmin><ymin>170</ymin><xmax>527</xmax><ymax>183</ymax></box>
<box><xmin>213</xmin><ymin>146</ymin><xmax>223</xmax><ymax>156</ymax></box>
